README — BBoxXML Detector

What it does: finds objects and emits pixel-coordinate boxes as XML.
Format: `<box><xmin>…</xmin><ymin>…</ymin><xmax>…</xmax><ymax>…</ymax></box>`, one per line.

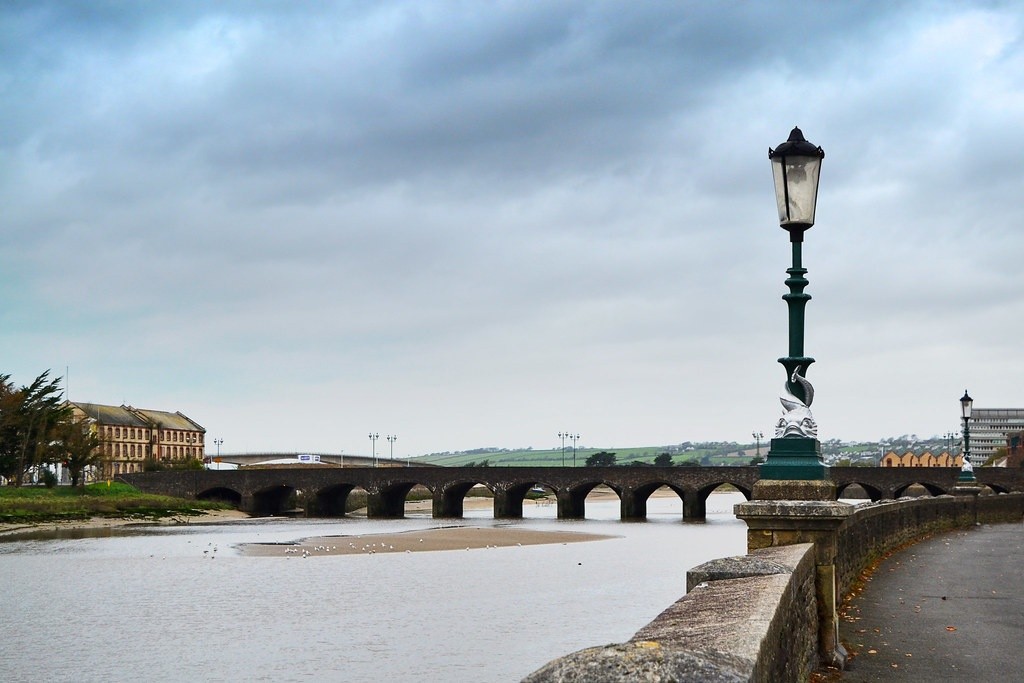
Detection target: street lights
<box><xmin>214</xmin><ymin>437</ymin><xmax>224</xmax><ymax>470</ymax></box>
<box><xmin>568</xmin><ymin>433</ymin><xmax>581</xmax><ymax>467</ymax></box>
<box><xmin>386</xmin><ymin>434</ymin><xmax>399</xmax><ymax>467</ymax></box>
<box><xmin>751</xmin><ymin>430</ymin><xmax>764</xmax><ymax>457</ymax></box>
<box><xmin>558</xmin><ymin>430</ymin><xmax>569</xmax><ymax>467</ymax></box>
<box><xmin>752</xmin><ymin>127</ymin><xmax>838</xmax><ymax>503</ymax></box>
<box><xmin>954</xmin><ymin>389</ymin><xmax>978</xmax><ymax>484</ymax></box>
<box><xmin>369</xmin><ymin>432</ymin><xmax>380</xmax><ymax>467</ymax></box>
